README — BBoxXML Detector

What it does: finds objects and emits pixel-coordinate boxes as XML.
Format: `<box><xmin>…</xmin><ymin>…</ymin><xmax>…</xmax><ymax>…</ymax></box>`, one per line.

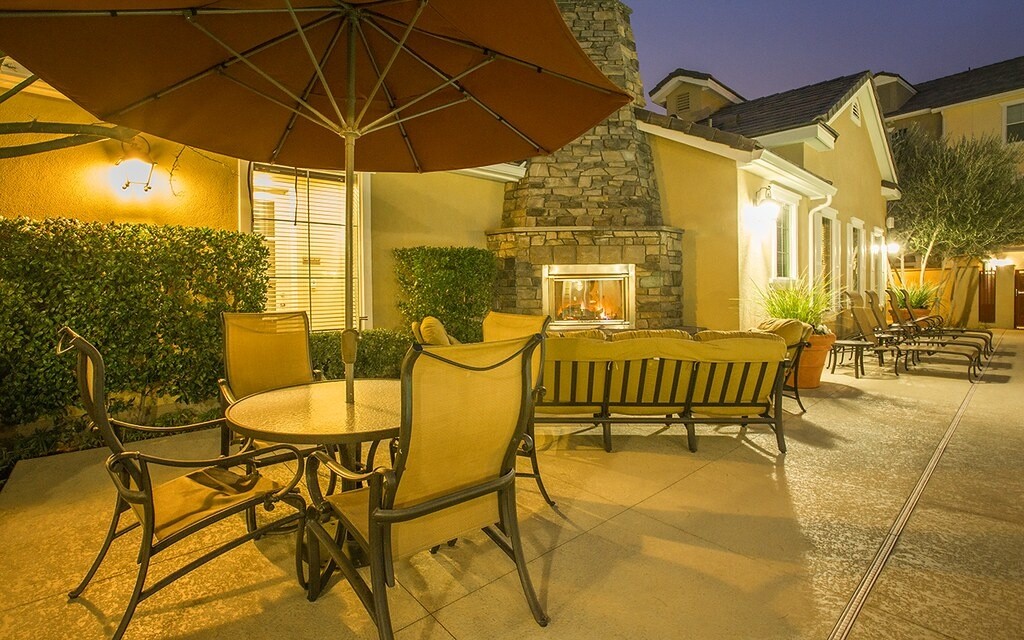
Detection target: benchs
<box><xmin>534</xmin><ymin>328</ymin><xmax>789</xmax><ymax>457</ymax></box>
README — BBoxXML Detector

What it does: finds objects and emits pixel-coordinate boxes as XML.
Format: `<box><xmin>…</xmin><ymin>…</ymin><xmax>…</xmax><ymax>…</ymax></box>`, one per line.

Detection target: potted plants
<box><xmin>730</xmin><ymin>263</ymin><xmax>860</xmax><ymax>391</ymax></box>
<box><xmin>885</xmin><ymin>278</ymin><xmax>952</xmax><ymax>328</ymax></box>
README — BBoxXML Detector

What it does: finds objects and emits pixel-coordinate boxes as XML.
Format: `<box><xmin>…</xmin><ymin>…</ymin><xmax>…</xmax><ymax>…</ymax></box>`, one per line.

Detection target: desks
<box><xmin>825</xmin><ymin>339</ymin><xmax>875</xmax><ymax>380</ymax></box>
<box><xmin>223</xmin><ymin>374</ymin><xmax>404</xmax><ymax>570</ymax></box>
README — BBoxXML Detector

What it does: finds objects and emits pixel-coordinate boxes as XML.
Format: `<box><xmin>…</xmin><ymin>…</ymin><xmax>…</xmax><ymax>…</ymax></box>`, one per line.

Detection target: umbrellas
<box><xmin>1</xmin><ymin>0</ymin><xmax>633</xmax><ymax>402</ymax></box>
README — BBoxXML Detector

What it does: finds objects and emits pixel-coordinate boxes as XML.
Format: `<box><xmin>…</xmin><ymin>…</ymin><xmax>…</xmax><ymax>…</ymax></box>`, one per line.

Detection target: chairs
<box><xmin>840</xmin><ymin>288</ymin><xmax>996</xmax><ymax>386</ymax></box>
<box><xmin>302</xmin><ymin>332</ymin><xmax>552</xmax><ymax>640</ymax></box>
<box><xmin>218</xmin><ymin>306</ymin><xmax>336</xmax><ymax>538</ymax></box>
<box><xmin>409</xmin><ymin>314</ymin><xmax>464</xmax><ymax>345</ymax></box>
<box><xmin>53</xmin><ymin>321</ymin><xmax>311</xmax><ymax>640</ymax></box>
<box><xmin>748</xmin><ymin>316</ymin><xmax>814</xmax><ymax>417</ymax></box>
<box><xmin>434</xmin><ymin>304</ymin><xmax>558</xmax><ymax>556</ymax></box>
